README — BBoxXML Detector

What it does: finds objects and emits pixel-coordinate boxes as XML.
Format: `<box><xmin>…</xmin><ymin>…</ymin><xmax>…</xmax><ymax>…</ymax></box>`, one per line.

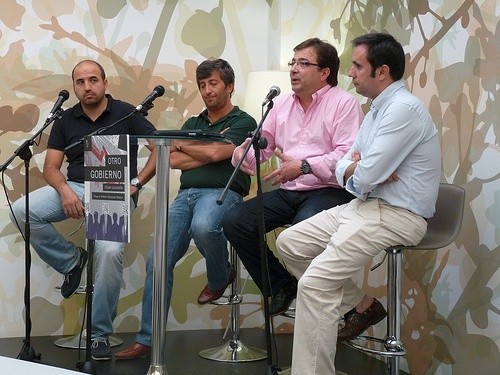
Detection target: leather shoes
<box><xmin>337</xmin><ymin>298</ymin><xmax>386</xmax><ymax>341</ymax></box>
<box><xmin>114</xmin><ymin>342</ymin><xmax>150</xmax><ymax>360</ymax></box>
<box><xmin>269</xmin><ymin>275</ymin><xmax>298</xmax><ymax>317</ymax></box>
<box><xmin>198</xmin><ymin>268</ymin><xmax>234</xmax><ymax>304</ymax></box>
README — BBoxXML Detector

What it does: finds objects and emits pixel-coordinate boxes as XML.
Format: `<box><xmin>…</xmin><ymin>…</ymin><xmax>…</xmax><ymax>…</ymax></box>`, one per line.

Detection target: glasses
<box><xmin>288</xmin><ymin>61</ymin><xmax>325</xmax><ymax>69</ymax></box>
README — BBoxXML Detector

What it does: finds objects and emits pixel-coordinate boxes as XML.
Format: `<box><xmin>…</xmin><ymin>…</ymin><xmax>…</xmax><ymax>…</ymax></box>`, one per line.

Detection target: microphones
<box><xmin>46</xmin><ymin>90</ymin><xmax>69</xmax><ymax>123</ymax></box>
<box><xmin>133</xmin><ymin>84</ymin><xmax>165</xmax><ymax>113</ymax></box>
<box><xmin>262</xmin><ymin>86</ymin><xmax>280</xmax><ymax>107</ymax></box>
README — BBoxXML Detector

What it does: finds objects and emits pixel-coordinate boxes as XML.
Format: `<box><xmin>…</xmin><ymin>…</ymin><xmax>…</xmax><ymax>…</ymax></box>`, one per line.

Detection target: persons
<box><xmin>10</xmin><ymin>61</ymin><xmax>159</xmax><ymax>359</ymax></box>
<box><xmin>275</xmin><ymin>33</ymin><xmax>441</xmax><ymax>374</ymax></box>
<box><xmin>115</xmin><ymin>58</ymin><xmax>257</xmax><ymax>360</ymax></box>
<box><xmin>226</xmin><ymin>39</ymin><xmax>365</xmax><ymax>313</ymax></box>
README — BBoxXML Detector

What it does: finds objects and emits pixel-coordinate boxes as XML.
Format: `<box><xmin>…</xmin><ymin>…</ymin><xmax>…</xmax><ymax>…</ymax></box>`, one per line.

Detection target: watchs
<box><xmin>302</xmin><ymin>159</ymin><xmax>310</xmax><ymax>174</ymax></box>
<box><xmin>130</xmin><ymin>178</ymin><xmax>142</xmax><ymax>190</ymax></box>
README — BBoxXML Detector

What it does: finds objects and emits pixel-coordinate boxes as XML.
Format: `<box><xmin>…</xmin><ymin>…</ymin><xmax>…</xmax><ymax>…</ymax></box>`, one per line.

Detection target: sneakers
<box><xmin>61</xmin><ymin>247</ymin><xmax>88</xmax><ymax>299</ymax></box>
<box><xmin>91</xmin><ymin>338</ymin><xmax>112</xmax><ymax>360</ymax></box>
